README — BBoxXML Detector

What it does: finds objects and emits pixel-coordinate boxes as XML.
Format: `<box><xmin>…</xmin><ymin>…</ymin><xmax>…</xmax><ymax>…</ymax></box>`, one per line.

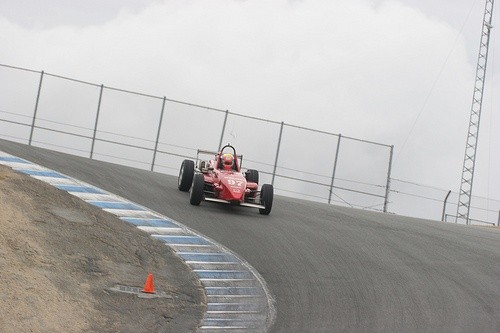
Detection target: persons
<box><xmin>218</xmin><ymin>154</ymin><xmax>237</xmax><ymax>172</ymax></box>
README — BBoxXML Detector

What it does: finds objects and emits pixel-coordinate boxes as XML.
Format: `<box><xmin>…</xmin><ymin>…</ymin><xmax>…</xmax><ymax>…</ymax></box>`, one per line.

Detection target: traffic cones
<box><xmin>141</xmin><ymin>274</ymin><xmax>157</xmax><ymax>294</ymax></box>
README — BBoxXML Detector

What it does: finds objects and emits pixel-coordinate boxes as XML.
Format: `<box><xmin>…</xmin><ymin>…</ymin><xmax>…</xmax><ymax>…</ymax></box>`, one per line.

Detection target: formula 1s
<box><xmin>177</xmin><ymin>144</ymin><xmax>274</xmax><ymax>215</ymax></box>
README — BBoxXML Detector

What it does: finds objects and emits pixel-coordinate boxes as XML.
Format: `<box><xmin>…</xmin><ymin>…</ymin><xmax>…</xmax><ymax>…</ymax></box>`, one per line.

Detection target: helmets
<box><xmin>222</xmin><ymin>154</ymin><xmax>235</xmax><ymax>171</ymax></box>
